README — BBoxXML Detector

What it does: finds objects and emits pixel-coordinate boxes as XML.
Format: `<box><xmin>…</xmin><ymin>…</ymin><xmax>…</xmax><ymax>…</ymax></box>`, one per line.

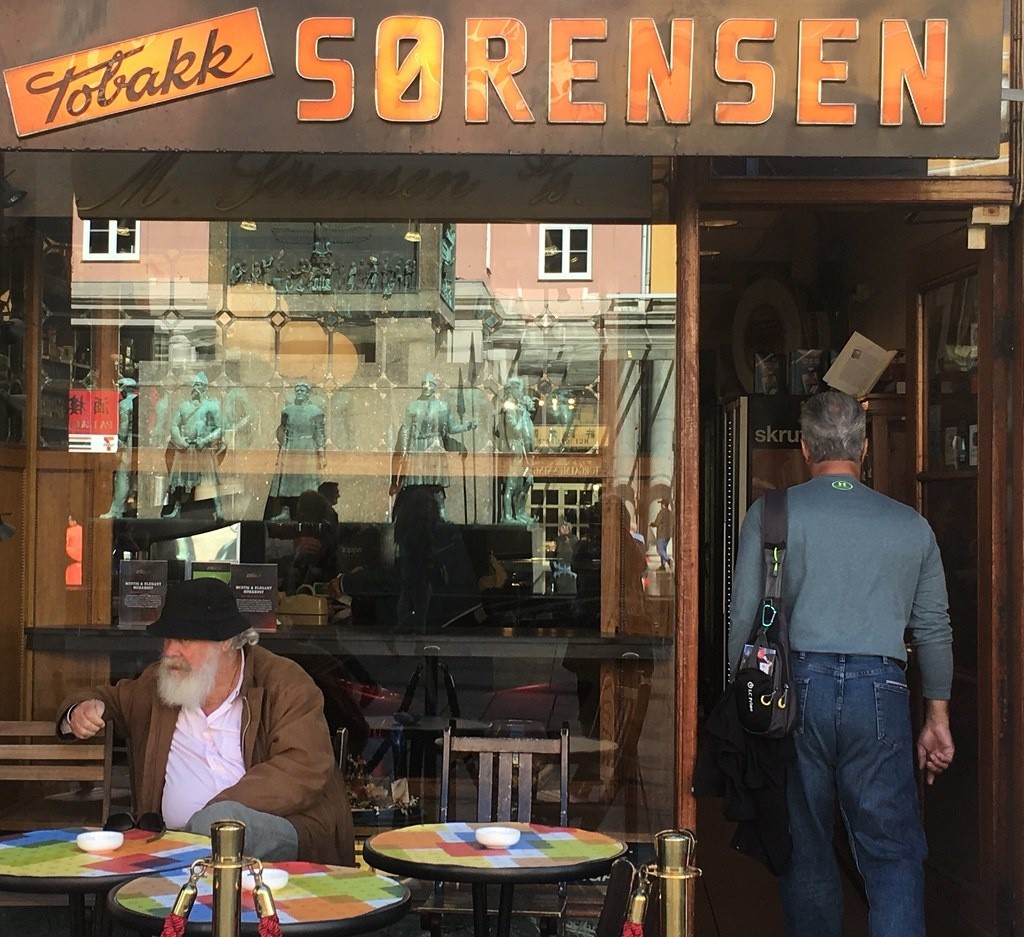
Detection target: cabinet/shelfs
<box><xmin>860</xmin><ymin>394</ymin><xmax>914</xmax><ymax>507</ymax></box>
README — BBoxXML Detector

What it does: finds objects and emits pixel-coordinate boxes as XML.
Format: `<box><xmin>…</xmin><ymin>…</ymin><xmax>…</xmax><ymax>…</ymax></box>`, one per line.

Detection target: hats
<box><xmin>145</xmin><ymin>578</ymin><xmax>251</xmax><ymax>642</ymax></box>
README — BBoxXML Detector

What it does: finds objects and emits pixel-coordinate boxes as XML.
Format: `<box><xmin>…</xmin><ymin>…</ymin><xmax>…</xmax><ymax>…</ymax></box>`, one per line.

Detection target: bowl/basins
<box><xmin>75</xmin><ymin>832</ymin><xmax>124</xmax><ymax>853</ymax></box>
<box><xmin>239</xmin><ymin>868</ymin><xmax>288</xmax><ymax>892</ymax></box>
<box><xmin>476</xmin><ymin>827</ymin><xmax>521</xmax><ymax>849</ymax></box>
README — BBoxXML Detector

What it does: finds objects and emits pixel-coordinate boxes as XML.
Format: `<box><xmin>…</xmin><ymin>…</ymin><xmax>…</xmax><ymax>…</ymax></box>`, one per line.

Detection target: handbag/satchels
<box><xmin>728</xmin><ymin>599</ymin><xmax>798</xmax><ymax>739</ymax></box>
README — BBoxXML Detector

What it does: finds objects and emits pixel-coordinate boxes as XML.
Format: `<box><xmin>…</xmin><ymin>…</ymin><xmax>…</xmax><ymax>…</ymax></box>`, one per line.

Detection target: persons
<box><xmin>288</xmin><ymin>573</ymin><xmax>372</xmax><ymax>757</ymax></box>
<box><xmin>317</xmin><ymin>479</ymin><xmax>340</xmax><ymax>506</ymax></box>
<box><xmin>99</xmin><ymin>377</ymin><xmax>137</xmax><ymax>519</ymax></box>
<box><xmin>646</xmin><ymin>498</ymin><xmax>674</xmax><ymax>569</ymax></box>
<box><xmin>395</xmin><ymin>373</ymin><xmax>477</xmax><ymax>521</ymax></box>
<box><xmin>266</xmin><ymin>381</ymin><xmax>327</xmax><ymax>521</ymax></box>
<box><xmin>554</xmin><ymin>521</ymin><xmax>578</xmax><ymax>594</ymax></box>
<box><xmin>295</xmin><ymin>487</ymin><xmax>339</xmax><ymax>575</ymax></box>
<box><xmin>497</xmin><ymin>380</ymin><xmax>539</xmax><ymax>525</ymax></box>
<box><xmin>263</xmin><ymin>522</ymin><xmax>321</xmax><ymax>564</ymax></box>
<box><xmin>463</xmin><ymin>528</ymin><xmax>508</xmax><ymax>624</ymax></box>
<box><xmin>228</xmin><ymin>240</ymin><xmax>416</xmax><ymax>297</ymax></box>
<box><xmin>727</xmin><ymin>391</ymin><xmax>956</xmax><ymax>937</ymax></box>
<box><xmin>53</xmin><ymin>578</ymin><xmax>356</xmax><ymax>868</ymax></box>
<box><xmin>161</xmin><ymin>372</ymin><xmax>230</xmax><ymax>523</ymax></box>
<box><xmin>391</xmin><ymin>493</ymin><xmax>443</xmax><ymax>637</ymax></box>
<box><xmin>329</xmin><ymin>557</ymin><xmax>394</xmax><ymax>605</ymax></box>
<box><xmin>561</xmin><ymin>498</ymin><xmax>655</xmax><ymax>798</ymax></box>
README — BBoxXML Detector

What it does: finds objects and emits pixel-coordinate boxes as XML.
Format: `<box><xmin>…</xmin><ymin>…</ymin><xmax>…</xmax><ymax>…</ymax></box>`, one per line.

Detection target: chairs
<box><xmin>0</xmin><ymin>718</ymin><xmax>113</xmax><ymax>909</ymax></box>
<box><xmin>534</xmin><ymin>669</ymin><xmax>653</xmax><ymax>833</ymax></box>
<box><xmin>433</xmin><ymin>723</ymin><xmax>569</xmax><ymax>936</ymax></box>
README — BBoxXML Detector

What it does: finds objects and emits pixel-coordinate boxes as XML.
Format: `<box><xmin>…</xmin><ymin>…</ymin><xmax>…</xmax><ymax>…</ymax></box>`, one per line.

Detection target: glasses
<box><xmin>102</xmin><ymin>811</ymin><xmax>166</xmax><ymax>843</ymax></box>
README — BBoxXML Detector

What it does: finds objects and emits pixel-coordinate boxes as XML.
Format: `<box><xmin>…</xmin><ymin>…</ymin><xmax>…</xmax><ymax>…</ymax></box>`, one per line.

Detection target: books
<box><xmin>751</xmin><ymin>343</ymin><xmax>884</xmax><ymax>398</ymax></box>
<box><xmin>822</xmin><ymin>331</ymin><xmax>898</xmax><ymax>397</ymax></box>
<box><xmin>856</xmin><ymin>362</ymin><xmax>905</xmax><ymax>403</ymax></box>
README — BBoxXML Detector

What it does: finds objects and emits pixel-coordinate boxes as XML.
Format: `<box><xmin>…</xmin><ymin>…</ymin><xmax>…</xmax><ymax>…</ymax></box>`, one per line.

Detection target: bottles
<box><xmin>955</xmin><ymin>413</ymin><xmax>969</xmax><ymax>465</ymax></box>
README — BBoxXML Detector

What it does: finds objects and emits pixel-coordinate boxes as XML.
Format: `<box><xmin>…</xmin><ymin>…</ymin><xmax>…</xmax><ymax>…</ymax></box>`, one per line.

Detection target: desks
<box><xmin>25</xmin><ymin>626</ymin><xmax>674</xmax><ymax>831</ymax></box>
<box><xmin>368</xmin><ymin>714</ymin><xmax>490</xmax><ymax>830</ymax></box>
<box><xmin>0</xmin><ymin>814</ymin><xmax>627</xmax><ymax>936</ymax></box>
